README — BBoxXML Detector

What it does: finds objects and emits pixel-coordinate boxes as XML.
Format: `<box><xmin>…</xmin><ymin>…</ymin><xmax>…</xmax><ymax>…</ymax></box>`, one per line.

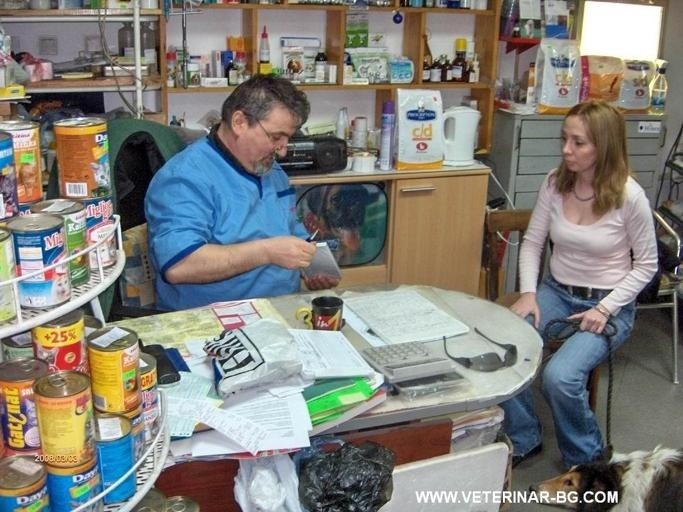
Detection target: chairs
<box><xmin>486</xmin><ymin>208</ymin><xmax>601</xmax><ymax>418</ymax></box>
<box><xmin>655</xmin><ymin>200</ymin><xmax>682</xmax><ymax>238</ymax></box>
<box><xmin>654</xmin><ymin>121</ymin><xmax>683</xmax><ymax>208</ymax></box>
<box><xmin>110</xmin><ymin>223</ymin><xmax>169</xmax><ymax>320</ymax></box>
<box><xmin>634</xmin><ymin>211</ymin><xmax>681</xmax><ymax>386</ymax></box>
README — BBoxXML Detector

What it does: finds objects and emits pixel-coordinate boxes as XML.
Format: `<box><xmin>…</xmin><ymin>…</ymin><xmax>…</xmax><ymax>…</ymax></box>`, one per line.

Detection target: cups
<box><xmin>24</xmin><ymin>64</ymin><xmax>40</xmax><ymax>82</ymax></box>
<box><xmin>295</xmin><ymin>295</ymin><xmax>346</xmax><ymax>331</ymax></box>
<box><xmin>42</xmin><ymin>63</ymin><xmax>53</xmax><ymax>80</ymax></box>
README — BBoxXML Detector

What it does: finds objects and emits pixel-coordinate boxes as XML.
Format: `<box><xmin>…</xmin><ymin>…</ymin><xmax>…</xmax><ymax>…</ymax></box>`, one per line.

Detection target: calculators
<box><xmin>365</xmin><ymin>341</ymin><xmax>452</xmax><ymax>383</ymax></box>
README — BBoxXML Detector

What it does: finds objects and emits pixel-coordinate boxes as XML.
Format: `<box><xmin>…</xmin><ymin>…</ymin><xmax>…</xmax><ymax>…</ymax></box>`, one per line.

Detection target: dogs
<box><xmin>527</xmin><ymin>446</ymin><xmax>681</xmax><ymax>512</ymax></box>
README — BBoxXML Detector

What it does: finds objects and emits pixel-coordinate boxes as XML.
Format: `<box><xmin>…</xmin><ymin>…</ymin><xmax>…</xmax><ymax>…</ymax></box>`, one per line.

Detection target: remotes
<box><xmin>142</xmin><ymin>344</ymin><xmax>178</xmax><ymax>381</ymax></box>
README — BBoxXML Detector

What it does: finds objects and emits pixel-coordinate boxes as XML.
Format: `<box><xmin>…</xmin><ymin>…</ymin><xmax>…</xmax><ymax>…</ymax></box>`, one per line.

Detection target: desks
<box><xmin>106</xmin><ymin>285</ymin><xmax>545</xmax><ymax>511</ymax></box>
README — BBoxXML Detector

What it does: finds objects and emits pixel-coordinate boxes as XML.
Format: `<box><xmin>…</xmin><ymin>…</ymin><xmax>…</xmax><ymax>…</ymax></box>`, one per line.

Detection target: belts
<box><xmin>560</xmin><ymin>284</ymin><xmax>614</xmax><ymax>300</ymax></box>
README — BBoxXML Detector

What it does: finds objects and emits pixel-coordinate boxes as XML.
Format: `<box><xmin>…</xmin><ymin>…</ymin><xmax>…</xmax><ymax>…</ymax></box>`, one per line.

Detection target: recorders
<box><xmin>277</xmin><ymin>129</ymin><xmax>347</xmax><ymax>176</ymax></box>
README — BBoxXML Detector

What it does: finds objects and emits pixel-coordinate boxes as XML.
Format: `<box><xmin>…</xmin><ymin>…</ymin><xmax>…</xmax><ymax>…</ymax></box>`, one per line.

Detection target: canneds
<box><xmin>32</xmin><ymin>370</ymin><xmax>94</xmax><ymax>469</ymax></box>
<box><xmin>84</xmin><ymin>196</ymin><xmax>117</xmax><ymax>271</ymax></box>
<box><xmin>0</xmin><ymin>225</ymin><xmax>19</xmax><ymax>325</ymax></box>
<box><xmin>0</xmin><ymin>358</ymin><xmax>49</xmax><ymax>451</ymax></box>
<box><xmin>2</xmin><ymin>333</ymin><xmax>34</xmax><ymax>359</ymax></box>
<box><xmin>34</xmin><ymin>198</ymin><xmax>90</xmax><ymax>289</ymax></box>
<box><xmin>95</xmin><ymin>411</ymin><xmax>137</xmax><ymax>504</ymax></box>
<box><xmin>55</xmin><ymin>117</ymin><xmax>113</xmax><ymax>199</ymax></box>
<box><xmin>31</xmin><ymin>309</ymin><xmax>88</xmax><ymax>372</ymax></box>
<box><xmin>125</xmin><ymin>407</ymin><xmax>146</xmax><ymax>469</ymax></box>
<box><xmin>14</xmin><ymin>200</ymin><xmax>38</xmax><ymax>213</ymax></box>
<box><xmin>45</xmin><ymin>455</ymin><xmax>104</xmax><ymax>512</ymax></box>
<box><xmin>2</xmin><ymin>120</ymin><xmax>43</xmax><ymax>201</ymax></box>
<box><xmin>0</xmin><ymin>454</ymin><xmax>49</xmax><ymax>512</ymax></box>
<box><xmin>84</xmin><ymin>314</ymin><xmax>102</xmax><ymax>338</ymax></box>
<box><xmin>0</xmin><ymin>132</ymin><xmax>20</xmax><ymax>222</ymax></box>
<box><xmin>86</xmin><ymin>327</ymin><xmax>140</xmax><ymax>414</ymax></box>
<box><xmin>6</xmin><ymin>212</ymin><xmax>71</xmax><ymax>310</ymax></box>
<box><xmin>140</xmin><ymin>353</ymin><xmax>158</xmax><ymax>412</ymax></box>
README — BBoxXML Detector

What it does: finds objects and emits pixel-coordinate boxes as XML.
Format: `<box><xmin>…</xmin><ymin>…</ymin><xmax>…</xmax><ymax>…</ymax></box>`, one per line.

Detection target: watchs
<box><xmin>592</xmin><ymin>305</ymin><xmax>611</xmax><ymax>321</ymax></box>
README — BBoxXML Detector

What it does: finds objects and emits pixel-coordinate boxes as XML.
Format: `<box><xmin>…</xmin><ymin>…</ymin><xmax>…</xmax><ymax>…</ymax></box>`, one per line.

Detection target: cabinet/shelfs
<box><xmin>486</xmin><ymin>110</ymin><xmax>668</xmax><ymax>295</ymax></box>
<box><xmin>166</xmin><ymin>1</ymin><xmax>505</xmax><ymax>155</ymax></box>
<box><xmin>0</xmin><ymin>0</ymin><xmax>169</xmax><ymax>128</ymax></box>
<box><xmin>0</xmin><ymin>214</ymin><xmax>171</xmax><ymax>512</ymax></box>
<box><xmin>287</xmin><ymin>161</ymin><xmax>493</xmax><ymax>295</ymax></box>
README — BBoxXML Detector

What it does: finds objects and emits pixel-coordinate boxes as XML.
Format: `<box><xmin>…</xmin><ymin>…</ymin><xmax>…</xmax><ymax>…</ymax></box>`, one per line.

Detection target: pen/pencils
<box><xmin>306</xmin><ymin>230</ymin><xmax>319</xmax><ymax>242</ymax></box>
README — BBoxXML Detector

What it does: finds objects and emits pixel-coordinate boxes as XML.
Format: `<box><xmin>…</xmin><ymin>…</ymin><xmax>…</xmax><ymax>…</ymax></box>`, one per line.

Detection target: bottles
<box><xmin>649</xmin><ymin>67</ymin><xmax>668</xmax><ymax>114</ymax></box>
<box><xmin>431</xmin><ymin>60</ymin><xmax>442</xmax><ymax>82</ymax></box>
<box><xmin>224</xmin><ymin>60</ymin><xmax>238</xmax><ymax>86</ymax></box>
<box><xmin>351</xmin><ymin>117</ymin><xmax>368</xmax><ymax>151</ymax></box>
<box><xmin>453</xmin><ymin>53</ymin><xmax>466</xmax><ymax>82</ymax></box>
<box><xmin>442</xmin><ymin>59</ymin><xmax>453</xmax><ymax>82</ymax></box>
<box><xmin>314</xmin><ymin>48</ymin><xmax>329</xmax><ymax>82</ymax></box>
<box><xmin>423</xmin><ymin>59</ymin><xmax>431</xmax><ymax>83</ymax></box>
<box><xmin>379</xmin><ymin>101</ymin><xmax>395</xmax><ymax>171</ymax></box>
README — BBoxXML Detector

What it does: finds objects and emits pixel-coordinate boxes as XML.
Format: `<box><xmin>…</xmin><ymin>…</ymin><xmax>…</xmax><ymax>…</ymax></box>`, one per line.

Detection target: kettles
<box><xmin>439</xmin><ymin>106</ymin><xmax>482</xmax><ymax>166</ymax></box>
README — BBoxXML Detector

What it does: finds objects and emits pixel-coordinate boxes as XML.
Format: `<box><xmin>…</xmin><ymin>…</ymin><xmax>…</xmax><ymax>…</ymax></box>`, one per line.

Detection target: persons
<box><xmin>497</xmin><ymin>105</ymin><xmax>658</xmax><ymax>474</ymax></box>
<box><xmin>144</xmin><ymin>73</ymin><xmax>341</xmax><ymax>314</ymax></box>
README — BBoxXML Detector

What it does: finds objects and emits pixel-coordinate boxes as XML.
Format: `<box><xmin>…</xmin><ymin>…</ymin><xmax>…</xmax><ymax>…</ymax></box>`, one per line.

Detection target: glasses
<box><xmin>257</xmin><ymin>119</ymin><xmax>296</xmax><ymax>151</ymax></box>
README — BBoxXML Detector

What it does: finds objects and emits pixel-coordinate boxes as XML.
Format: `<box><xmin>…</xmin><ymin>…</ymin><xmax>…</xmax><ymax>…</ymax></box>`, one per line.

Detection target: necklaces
<box><xmin>573</xmin><ymin>190</ymin><xmax>595</xmax><ymax>202</ymax></box>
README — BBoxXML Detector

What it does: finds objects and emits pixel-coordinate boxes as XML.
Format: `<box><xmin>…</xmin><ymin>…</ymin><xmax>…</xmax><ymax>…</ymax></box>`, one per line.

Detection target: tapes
<box><xmin>346</xmin><ymin>152</ymin><xmax>376</xmax><ymax>174</ymax></box>
